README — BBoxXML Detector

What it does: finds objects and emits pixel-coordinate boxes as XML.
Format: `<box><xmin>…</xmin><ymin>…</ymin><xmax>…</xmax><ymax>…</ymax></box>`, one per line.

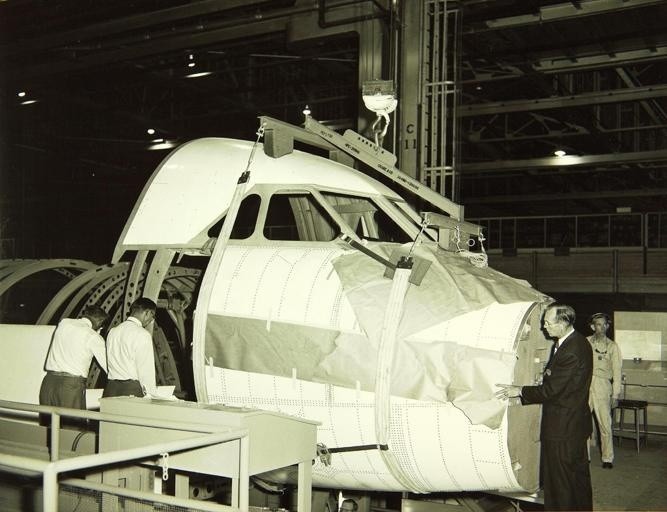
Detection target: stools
<box><xmin>614</xmin><ymin>399</ymin><xmax>648</xmax><ymax>454</ymax></box>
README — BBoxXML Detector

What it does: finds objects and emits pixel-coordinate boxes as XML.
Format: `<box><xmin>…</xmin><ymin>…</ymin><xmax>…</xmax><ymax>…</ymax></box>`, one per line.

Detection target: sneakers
<box><xmin>602</xmin><ymin>462</ymin><xmax>613</xmax><ymax>470</ymax></box>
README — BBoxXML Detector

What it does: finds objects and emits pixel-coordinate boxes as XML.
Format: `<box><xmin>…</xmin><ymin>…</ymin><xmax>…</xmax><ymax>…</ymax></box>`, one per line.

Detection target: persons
<box><xmin>494</xmin><ymin>301</ymin><xmax>593</xmax><ymax>511</ymax></box>
<box><xmin>39</xmin><ymin>305</ymin><xmax>108</xmax><ymax>431</ymax></box>
<box><xmin>102</xmin><ymin>297</ymin><xmax>158</xmax><ymax>399</ymax></box>
<box><xmin>585</xmin><ymin>312</ymin><xmax>622</xmax><ymax>468</ymax></box>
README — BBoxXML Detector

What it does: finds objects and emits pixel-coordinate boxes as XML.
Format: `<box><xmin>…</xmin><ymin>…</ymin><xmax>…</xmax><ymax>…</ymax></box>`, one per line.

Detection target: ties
<box><xmin>553</xmin><ymin>341</ymin><xmax>559</xmax><ymax>355</ymax></box>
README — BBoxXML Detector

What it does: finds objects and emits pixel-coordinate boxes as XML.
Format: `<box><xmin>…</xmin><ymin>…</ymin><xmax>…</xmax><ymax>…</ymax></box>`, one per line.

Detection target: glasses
<box><xmin>146</xmin><ymin>310</ymin><xmax>158</xmax><ymax>321</ymax></box>
<box><xmin>544</xmin><ymin>320</ymin><xmax>559</xmax><ymax>327</ymax></box>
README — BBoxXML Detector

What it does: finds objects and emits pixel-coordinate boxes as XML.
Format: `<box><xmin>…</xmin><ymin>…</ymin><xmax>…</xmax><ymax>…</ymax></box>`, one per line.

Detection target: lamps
<box><xmin>183</xmin><ymin>53</ymin><xmax>213</xmax><ymax>80</ymax></box>
<box><xmin>146</xmin><ymin>123</ymin><xmax>175</xmax><ymax>154</ymax></box>
